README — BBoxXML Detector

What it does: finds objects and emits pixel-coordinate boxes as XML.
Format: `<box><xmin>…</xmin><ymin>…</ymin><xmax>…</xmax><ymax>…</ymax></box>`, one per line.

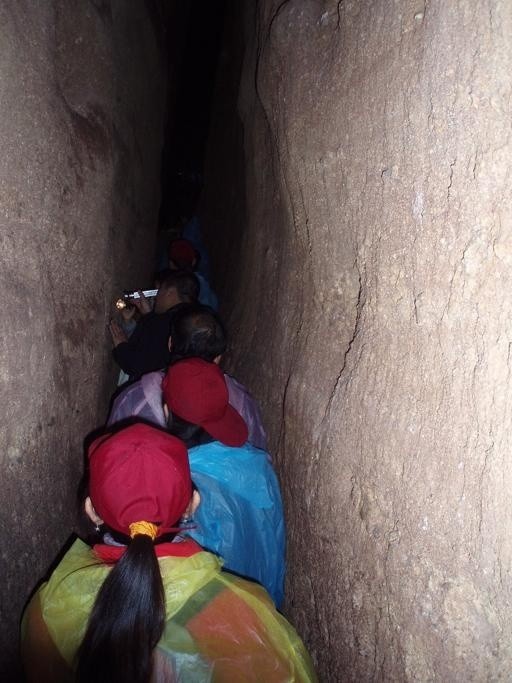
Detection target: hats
<box><xmin>89</xmin><ymin>423</ymin><xmax>196</xmax><ymax>535</ymax></box>
<box><xmin>162</xmin><ymin>359</ymin><xmax>248</xmax><ymax>446</ymax></box>
<box><xmin>170</xmin><ymin>239</ymin><xmax>195</xmax><ymax>262</ymax></box>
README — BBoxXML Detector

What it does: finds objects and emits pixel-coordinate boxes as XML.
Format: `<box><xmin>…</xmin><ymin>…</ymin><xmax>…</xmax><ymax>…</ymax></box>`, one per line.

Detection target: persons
<box><xmin>156</xmin><ymin>357</ymin><xmax>287</xmax><ymax>613</ymax></box>
<box><xmin>15</xmin><ymin>419</ymin><xmax>318</xmax><ymax>683</ymax></box>
<box><xmin>103</xmin><ymin>301</ymin><xmax>271</xmax><ymax>453</ymax></box>
<box><xmin>165</xmin><ymin>239</ymin><xmax>218</xmax><ymax>312</ymax></box>
<box><xmin>107</xmin><ymin>267</ymin><xmax>201</xmax><ymax>381</ymax></box>
